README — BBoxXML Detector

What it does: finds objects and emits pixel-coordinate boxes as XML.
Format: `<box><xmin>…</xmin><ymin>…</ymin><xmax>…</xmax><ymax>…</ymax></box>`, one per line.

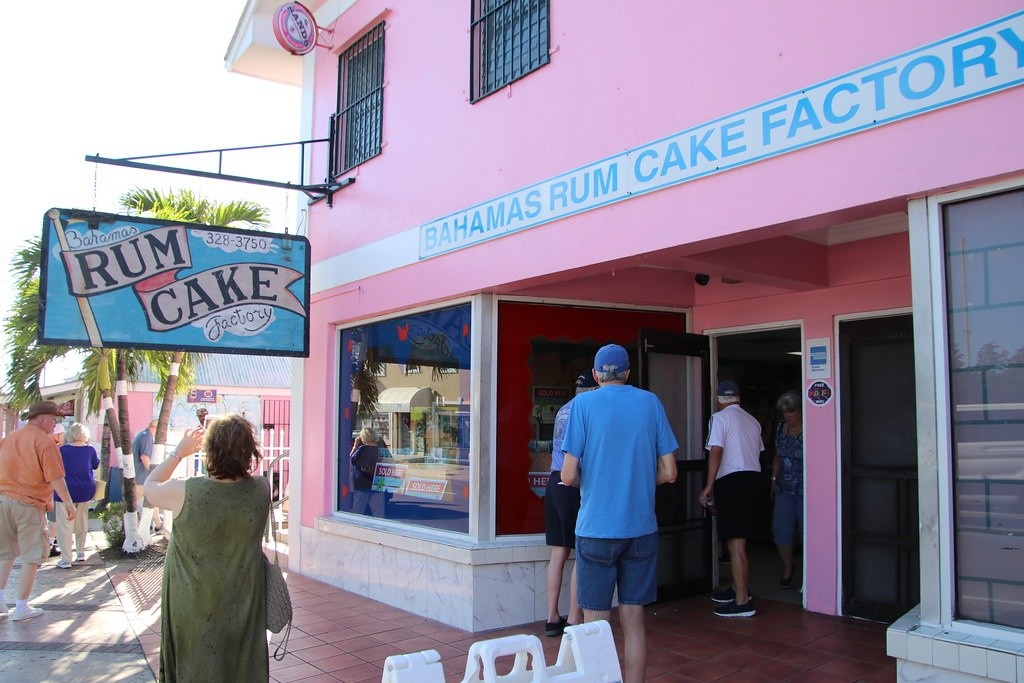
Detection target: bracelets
<box><xmin>772</xmin><ymin>477</ymin><xmax>776</xmax><ymax>481</ymax></box>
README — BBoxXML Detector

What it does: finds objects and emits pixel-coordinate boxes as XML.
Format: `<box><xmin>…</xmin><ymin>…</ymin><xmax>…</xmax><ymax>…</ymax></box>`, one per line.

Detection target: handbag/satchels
<box><xmin>263</xmin><ymin>553</ymin><xmax>292</xmax><ymax>634</ymax></box>
<box><xmin>91</xmin><ymin>478</ymin><xmax>107</xmax><ymax>500</ymax></box>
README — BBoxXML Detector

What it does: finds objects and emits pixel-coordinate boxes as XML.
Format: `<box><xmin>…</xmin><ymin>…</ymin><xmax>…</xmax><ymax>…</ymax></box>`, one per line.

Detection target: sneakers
<box><xmin>713</xmin><ymin>600</ymin><xmax>756</xmax><ymax>616</ymax></box>
<box><xmin>13</xmin><ymin>607</ymin><xmax>43</xmax><ymax>620</ymax></box>
<box><xmin>0</xmin><ymin>602</ymin><xmax>8</xmax><ymax>613</ymax></box>
<box><xmin>711</xmin><ymin>585</ymin><xmax>752</xmax><ymax>602</ymax></box>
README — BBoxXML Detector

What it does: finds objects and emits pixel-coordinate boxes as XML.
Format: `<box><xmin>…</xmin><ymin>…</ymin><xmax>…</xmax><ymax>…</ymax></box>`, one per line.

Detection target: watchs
<box><xmin>170</xmin><ymin>450</ymin><xmax>182</xmax><ymax>461</ymax></box>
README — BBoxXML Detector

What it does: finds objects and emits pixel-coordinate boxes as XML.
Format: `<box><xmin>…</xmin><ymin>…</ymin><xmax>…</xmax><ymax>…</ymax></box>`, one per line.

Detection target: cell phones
<box><xmin>204</xmin><ymin>415</ymin><xmax>213</xmax><ymax>430</ymax></box>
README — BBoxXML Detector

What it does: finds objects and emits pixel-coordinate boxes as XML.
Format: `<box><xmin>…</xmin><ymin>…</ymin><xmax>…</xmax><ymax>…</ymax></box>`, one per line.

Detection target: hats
<box><xmin>576</xmin><ymin>370</ymin><xmax>601</xmax><ymax>387</ymax></box>
<box><xmin>21</xmin><ymin>400</ymin><xmax>65</xmax><ymax>421</ymax></box>
<box><xmin>594</xmin><ymin>344</ymin><xmax>629</xmax><ymax>372</ymax></box>
<box><xmin>717</xmin><ymin>381</ymin><xmax>740</xmax><ymax>396</ymax></box>
<box><xmin>53</xmin><ymin>423</ymin><xmax>66</xmax><ymax>433</ymax></box>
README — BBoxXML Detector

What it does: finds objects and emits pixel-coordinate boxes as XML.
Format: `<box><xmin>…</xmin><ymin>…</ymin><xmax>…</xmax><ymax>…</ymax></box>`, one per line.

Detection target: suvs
<box><xmin>351</xmin><ymin>430</ymin><xmax>397</xmax><ymax>503</ymax></box>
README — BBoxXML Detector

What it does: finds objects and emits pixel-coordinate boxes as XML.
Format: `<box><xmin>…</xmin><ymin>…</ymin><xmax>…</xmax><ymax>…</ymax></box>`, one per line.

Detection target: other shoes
<box><xmin>52</xmin><ymin>537</ymin><xmax>57</xmax><ymax>545</ymax></box>
<box><xmin>544</xmin><ymin>616</ymin><xmax>567</xmax><ymax>636</ymax></box>
<box><xmin>77</xmin><ymin>555</ymin><xmax>85</xmax><ymax>561</ymax></box>
<box><xmin>780</xmin><ymin>575</ymin><xmax>793</xmax><ymax>588</ymax></box>
<box><xmin>58</xmin><ymin>560</ymin><xmax>71</xmax><ymax>569</ymax></box>
<box><xmin>48</xmin><ymin>545</ymin><xmax>61</xmax><ymax>558</ymax></box>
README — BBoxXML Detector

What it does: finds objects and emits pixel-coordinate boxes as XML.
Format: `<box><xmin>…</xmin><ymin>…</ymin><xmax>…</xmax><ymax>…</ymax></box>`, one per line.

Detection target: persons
<box><xmin>700</xmin><ymin>380</ymin><xmax>765</xmax><ymax>616</ymax></box>
<box><xmin>350</xmin><ymin>427</ymin><xmax>385</xmax><ymax>518</ymax></box>
<box><xmin>770</xmin><ymin>393</ymin><xmax>803</xmax><ymax>587</ymax></box>
<box><xmin>545</xmin><ymin>370</ymin><xmax>600</xmax><ymax>635</ymax></box>
<box><xmin>144</xmin><ymin>414</ymin><xmax>271</xmax><ymax>683</ymax></box>
<box><xmin>560</xmin><ymin>343</ymin><xmax>679</xmax><ymax>683</ymax></box>
<box><xmin>54</xmin><ymin>422</ymin><xmax>100</xmax><ymax>568</ymax></box>
<box><xmin>46</xmin><ymin>423</ymin><xmax>66</xmax><ymax>557</ymax></box>
<box><xmin>133</xmin><ymin>418</ymin><xmax>163</xmax><ymax>533</ymax></box>
<box><xmin>0</xmin><ymin>401</ymin><xmax>77</xmax><ymax>620</ymax></box>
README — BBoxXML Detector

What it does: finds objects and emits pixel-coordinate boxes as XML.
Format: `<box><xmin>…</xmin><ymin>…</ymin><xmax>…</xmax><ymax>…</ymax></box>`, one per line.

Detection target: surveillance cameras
<box><xmin>695</xmin><ymin>274</ymin><xmax>710</xmax><ymax>286</ymax></box>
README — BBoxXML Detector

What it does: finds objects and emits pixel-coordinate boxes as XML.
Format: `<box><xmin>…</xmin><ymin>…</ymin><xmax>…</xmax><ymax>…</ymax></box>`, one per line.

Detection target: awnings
<box><xmin>375</xmin><ymin>387</ymin><xmax>432</xmax><ymax>413</ymax></box>
